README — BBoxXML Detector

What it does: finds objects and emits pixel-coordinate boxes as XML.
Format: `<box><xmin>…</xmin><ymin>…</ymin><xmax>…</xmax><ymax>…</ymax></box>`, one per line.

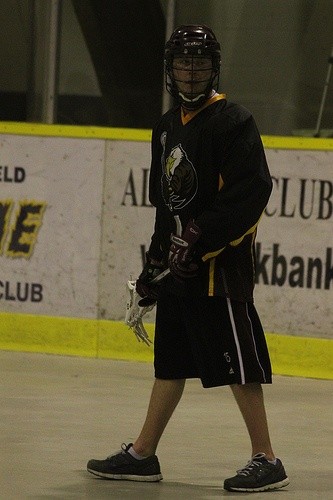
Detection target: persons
<box><xmin>84</xmin><ymin>21</ymin><xmax>292</xmax><ymax>494</ymax></box>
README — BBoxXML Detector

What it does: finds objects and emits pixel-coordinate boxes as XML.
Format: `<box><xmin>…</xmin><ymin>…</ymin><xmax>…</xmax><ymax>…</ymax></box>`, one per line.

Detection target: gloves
<box><xmin>135</xmin><ymin>252</ymin><xmax>173</xmax><ymax>307</ymax></box>
<box><xmin>168</xmin><ymin>222</ymin><xmax>204</xmax><ymax>283</ymax></box>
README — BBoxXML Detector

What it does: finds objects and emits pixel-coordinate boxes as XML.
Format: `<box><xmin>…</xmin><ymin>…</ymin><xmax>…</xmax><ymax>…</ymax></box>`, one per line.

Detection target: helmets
<box><xmin>165</xmin><ymin>24</ymin><xmax>220</xmax><ymax>59</ymax></box>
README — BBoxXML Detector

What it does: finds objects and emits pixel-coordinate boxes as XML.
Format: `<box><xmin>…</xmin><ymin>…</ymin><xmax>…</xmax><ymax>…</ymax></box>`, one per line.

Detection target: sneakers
<box><xmin>86</xmin><ymin>443</ymin><xmax>163</xmax><ymax>482</ymax></box>
<box><xmin>224</xmin><ymin>452</ymin><xmax>289</xmax><ymax>492</ymax></box>
